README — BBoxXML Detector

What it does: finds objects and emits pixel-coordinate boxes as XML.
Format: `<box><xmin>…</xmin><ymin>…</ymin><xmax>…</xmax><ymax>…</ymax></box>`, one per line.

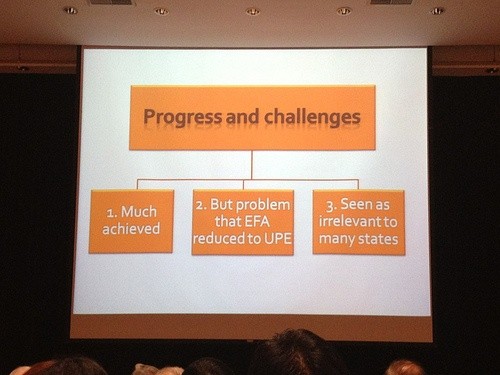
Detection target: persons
<box><xmin>246</xmin><ymin>330</ymin><xmax>350</xmax><ymax>374</ymax></box>
<box><xmin>157</xmin><ymin>367</ymin><xmax>184</xmax><ymax>375</ymax></box>
<box><xmin>9</xmin><ymin>365</ymin><xmax>32</xmax><ymax>374</ymax></box>
<box><xmin>385</xmin><ymin>359</ymin><xmax>424</xmax><ymax>375</ymax></box>
<box><xmin>181</xmin><ymin>357</ymin><xmax>234</xmax><ymax>375</ymax></box>
<box><xmin>133</xmin><ymin>363</ymin><xmax>159</xmax><ymax>375</ymax></box>
<box><xmin>23</xmin><ymin>360</ymin><xmax>60</xmax><ymax>375</ymax></box>
<box><xmin>40</xmin><ymin>357</ymin><xmax>106</xmax><ymax>375</ymax></box>
